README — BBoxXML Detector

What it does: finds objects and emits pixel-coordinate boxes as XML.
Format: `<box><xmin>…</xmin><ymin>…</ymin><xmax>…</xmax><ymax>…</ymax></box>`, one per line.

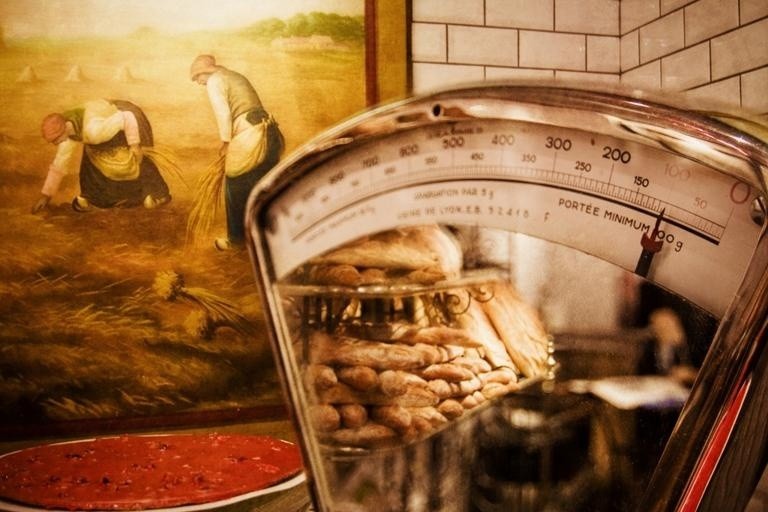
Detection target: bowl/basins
<box><xmin>0</xmin><ymin>434</ymin><xmax>308</xmax><ymax>512</ymax></box>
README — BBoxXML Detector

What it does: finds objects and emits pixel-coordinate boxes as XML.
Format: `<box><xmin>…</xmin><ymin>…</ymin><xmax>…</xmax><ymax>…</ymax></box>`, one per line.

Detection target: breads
<box><xmin>281</xmin><ymin>225</ymin><xmax>555</xmax><ymax>447</ymax></box>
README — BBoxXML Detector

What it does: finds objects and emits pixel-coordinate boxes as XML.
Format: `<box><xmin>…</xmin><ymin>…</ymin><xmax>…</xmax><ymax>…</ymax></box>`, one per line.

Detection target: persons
<box><xmin>190</xmin><ymin>54</ymin><xmax>285</xmax><ymax>250</ymax></box>
<box><xmin>33</xmin><ymin>98</ymin><xmax>172</xmax><ymax>214</ymax></box>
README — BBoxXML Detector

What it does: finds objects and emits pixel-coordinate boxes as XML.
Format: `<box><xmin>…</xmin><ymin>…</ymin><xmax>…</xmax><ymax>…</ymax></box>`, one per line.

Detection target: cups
<box><xmin>474</xmin><ymin>392</ymin><xmax>602</xmax><ymax>510</ymax></box>
